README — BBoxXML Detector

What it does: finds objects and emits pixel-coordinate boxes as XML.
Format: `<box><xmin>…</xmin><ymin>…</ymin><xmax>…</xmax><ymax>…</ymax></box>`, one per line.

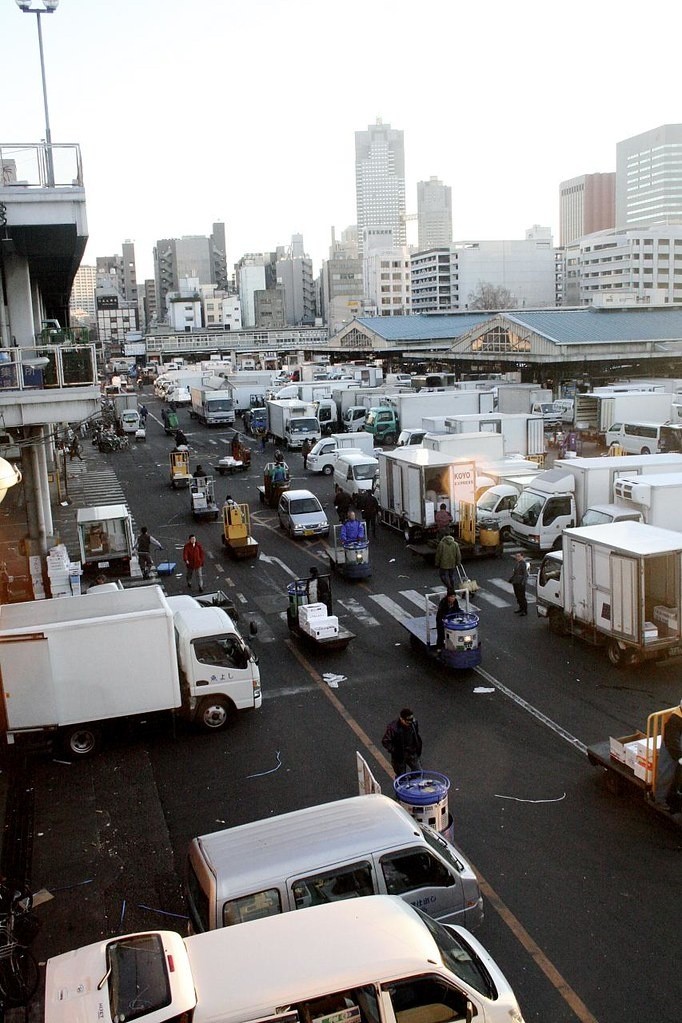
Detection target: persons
<box><xmin>133</xmin><ymin>527</ymin><xmax>164</xmax><ymax>580</ymax></box>
<box><xmin>257</xmin><ymin>425</ymin><xmax>266</xmax><ymax>454</ymax></box>
<box><xmin>509</xmin><ymin>551</ymin><xmax>529</xmax><ymax>617</ymax></box>
<box><xmin>193</xmin><ymin>465</ymin><xmax>207</xmax><ymax>486</ymax></box>
<box><xmin>382</xmin><ymin>708</ymin><xmax>422</xmax><ymax>779</ymax></box>
<box><xmin>141</xmin><ymin>406</ymin><xmax>148</xmax><ymax>423</ymax></box>
<box><xmin>271</xmin><ymin>450</ymin><xmax>287</xmax><ymax>488</ymax></box>
<box><xmin>223</xmin><ymin>495</ymin><xmax>244</xmax><ymax>518</ymax></box>
<box><xmin>654</xmin><ymin>700</ymin><xmax>682</xmax><ymax>813</ymax></box>
<box><xmin>89</xmin><ymin>575</ymin><xmax>106</xmax><ymax>588</ymax></box>
<box><xmin>161</xmin><ymin>401</ymin><xmax>177</xmax><ymax>428</ymax></box>
<box><xmin>231</xmin><ymin>433</ymin><xmax>239</xmax><ymax>451</ymax></box>
<box><xmin>435</xmin><ymin>503</ymin><xmax>461</xmax><ymax>589</ymax></box>
<box><xmin>427</xmin><ymin>474</ymin><xmax>444</xmax><ymax>502</ymax></box>
<box><xmin>436</xmin><ymin>587</ymin><xmax>461</xmax><ymax>654</ymax></box>
<box><xmin>334</xmin><ymin>487</ymin><xmax>378</xmax><ymax>545</ymax></box>
<box><xmin>175</xmin><ymin>430</ymin><xmax>189</xmax><ymax>447</ymax></box>
<box><xmin>70</xmin><ymin>436</ymin><xmax>83</xmax><ymax>462</ymax></box>
<box><xmin>306</xmin><ymin>567</ymin><xmax>332</xmax><ymax>616</ymax></box>
<box><xmin>183</xmin><ymin>535</ymin><xmax>205</xmax><ymax>591</ymax></box>
<box><xmin>302</xmin><ymin>437</ymin><xmax>317</xmax><ymax>470</ymax></box>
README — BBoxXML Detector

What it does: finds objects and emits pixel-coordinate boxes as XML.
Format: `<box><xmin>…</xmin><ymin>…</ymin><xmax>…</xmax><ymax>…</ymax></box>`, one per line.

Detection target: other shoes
<box><xmin>514</xmin><ymin>608</ymin><xmax>522</xmax><ymax>613</ymax></box>
<box><xmin>520</xmin><ymin>612</ymin><xmax>528</xmax><ymax>617</ymax></box>
<box><xmin>199</xmin><ymin>587</ymin><xmax>204</xmax><ymax>592</ymax></box>
<box><xmin>188</xmin><ymin>582</ymin><xmax>192</xmax><ymax>587</ymax></box>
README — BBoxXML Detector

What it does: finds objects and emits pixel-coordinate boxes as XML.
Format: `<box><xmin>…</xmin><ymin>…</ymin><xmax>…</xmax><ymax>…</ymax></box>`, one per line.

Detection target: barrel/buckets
<box><xmin>344</xmin><ymin>539</ymin><xmax>370</xmax><ymax>564</ymax></box>
<box><xmin>394</xmin><ymin>770</ymin><xmax>450</xmax><ymax>832</ymax></box>
<box><xmin>287</xmin><ymin>580</ymin><xmax>309</xmax><ymax>617</ymax></box>
<box><xmin>443</xmin><ymin>612</ymin><xmax>480</xmax><ymax>651</ymax></box>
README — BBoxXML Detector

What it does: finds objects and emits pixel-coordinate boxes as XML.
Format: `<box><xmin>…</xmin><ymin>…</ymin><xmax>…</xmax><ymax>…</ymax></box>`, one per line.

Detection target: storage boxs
<box><xmin>298</xmin><ymin>602</ymin><xmax>338</xmax><ymax>639</ymax></box>
<box><xmin>192</xmin><ymin>492</ymin><xmax>207</xmax><ymax>508</ymax></box>
<box><xmin>608</xmin><ymin>729</ymin><xmax>662</xmax><ymax>784</ymax></box>
<box><xmin>645</xmin><ymin>606</ymin><xmax>678</xmax><ymax>639</ymax></box>
<box><xmin>46</xmin><ymin>544</ymin><xmax>81</xmax><ymax>598</ymax></box>
<box><xmin>312</xmin><ymin>1005</ymin><xmax>361</xmax><ymax>1023</ymax></box>
<box><xmin>436</xmin><ymin>499</ymin><xmax>450</xmax><ymax>512</ymax></box>
<box><xmin>136</xmin><ymin>430</ymin><xmax>145</xmax><ymax>437</ymax></box>
<box><xmin>219</xmin><ymin>456</ymin><xmax>234</xmax><ymax>465</ymax></box>
<box><xmin>425</xmin><ymin>499</ymin><xmax>435</xmax><ymax>526</ymax></box>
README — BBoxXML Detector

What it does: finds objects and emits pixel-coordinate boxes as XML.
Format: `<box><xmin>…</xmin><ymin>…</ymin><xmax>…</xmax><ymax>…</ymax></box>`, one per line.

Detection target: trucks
<box><xmin>0</xmin><ymin>584</ymin><xmax>263</xmax><ymax>763</ymax></box>
<box><xmin>44</xmin><ymin>895</ymin><xmax>525</xmax><ymax>1023</ymax></box>
<box><xmin>536</xmin><ymin>520</ymin><xmax>682</xmax><ymax>666</ymax></box>
<box><xmin>104</xmin><ymin>357</ymin><xmax>682</xmax><ymax>556</ymax></box>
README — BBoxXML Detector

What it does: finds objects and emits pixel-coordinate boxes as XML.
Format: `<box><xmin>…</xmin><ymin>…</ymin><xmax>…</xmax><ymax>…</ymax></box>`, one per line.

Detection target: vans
<box><xmin>331</xmin><ymin>448</ymin><xmax>379</xmax><ymax>497</ymax></box>
<box><xmin>118</xmin><ymin>408</ymin><xmax>140</xmax><ymax>433</ymax></box>
<box><xmin>278</xmin><ymin>489</ymin><xmax>331</xmax><ymax>541</ymax></box>
<box><xmin>186</xmin><ymin>793</ymin><xmax>485</xmax><ymax>932</ymax></box>
